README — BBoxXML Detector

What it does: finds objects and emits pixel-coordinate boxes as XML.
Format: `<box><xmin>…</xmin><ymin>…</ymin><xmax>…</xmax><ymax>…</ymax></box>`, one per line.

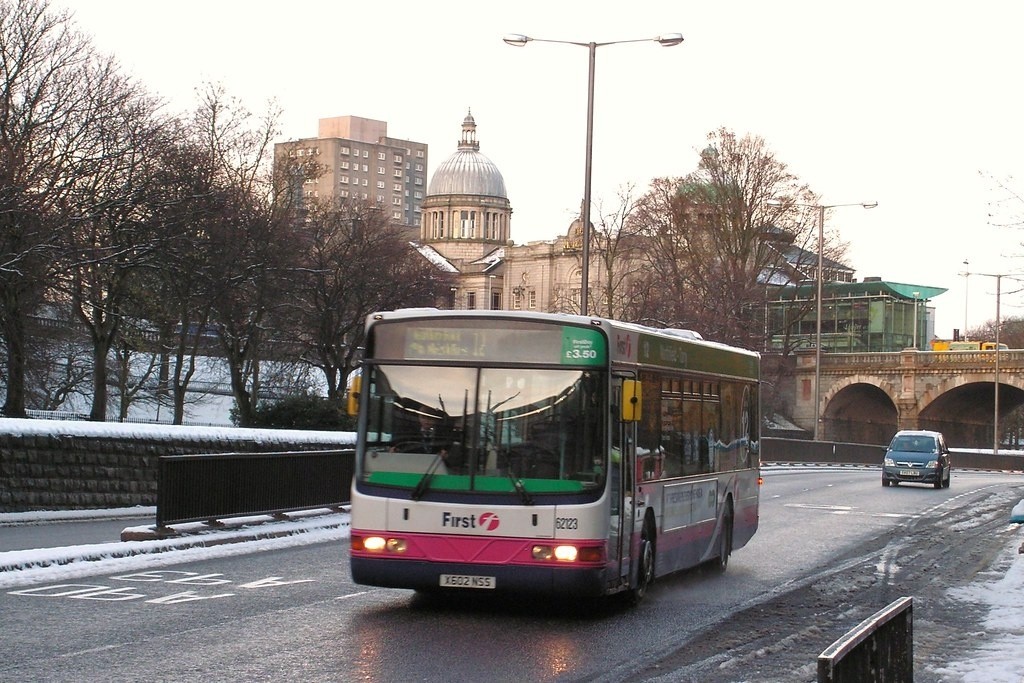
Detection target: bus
<box><xmin>347</xmin><ymin>308</ymin><xmax>761</xmax><ymax>604</ymax></box>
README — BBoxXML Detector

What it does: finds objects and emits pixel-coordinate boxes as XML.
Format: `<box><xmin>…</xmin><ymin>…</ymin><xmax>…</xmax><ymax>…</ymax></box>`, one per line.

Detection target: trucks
<box><xmin>933</xmin><ymin>342</ymin><xmax>1009</xmax><ymax>351</ymax></box>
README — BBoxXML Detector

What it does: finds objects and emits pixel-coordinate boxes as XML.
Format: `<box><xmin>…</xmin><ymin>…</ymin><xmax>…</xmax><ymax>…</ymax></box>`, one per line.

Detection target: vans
<box><xmin>881</xmin><ymin>429</ymin><xmax>951</xmax><ymax>489</ymax></box>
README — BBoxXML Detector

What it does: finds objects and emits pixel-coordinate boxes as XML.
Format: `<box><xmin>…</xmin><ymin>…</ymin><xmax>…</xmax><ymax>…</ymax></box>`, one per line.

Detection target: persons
<box><xmin>390</xmin><ymin>407</ymin><xmax>450</xmax><ymax>459</ymax></box>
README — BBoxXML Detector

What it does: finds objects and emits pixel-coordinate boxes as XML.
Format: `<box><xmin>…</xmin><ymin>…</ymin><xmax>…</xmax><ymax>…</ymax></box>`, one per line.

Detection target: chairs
<box><xmin>923</xmin><ymin>440</ymin><xmax>935</xmax><ymax>453</ymax></box>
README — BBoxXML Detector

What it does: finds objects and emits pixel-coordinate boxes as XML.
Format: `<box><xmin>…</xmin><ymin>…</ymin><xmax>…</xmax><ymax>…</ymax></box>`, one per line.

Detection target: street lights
<box><xmin>913</xmin><ymin>292</ymin><xmax>919</xmax><ymax>349</ymax></box>
<box><xmin>958</xmin><ymin>272</ymin><xmax>1024</xmax><ymax>455</ymax></box>
<box><xmin>502</xmin><ymin>33</ymin><xmax>683</xmax><ymax>318</ymax></box>
<box><xmin>962</xmin><ymin>258</ymin><xmax>969</xmax><ymax>341</ymax></box>
<box><xmin>765</xmin><ymin>199</ymin><xmax>878</xmax><ymax>441</ymax></box>
